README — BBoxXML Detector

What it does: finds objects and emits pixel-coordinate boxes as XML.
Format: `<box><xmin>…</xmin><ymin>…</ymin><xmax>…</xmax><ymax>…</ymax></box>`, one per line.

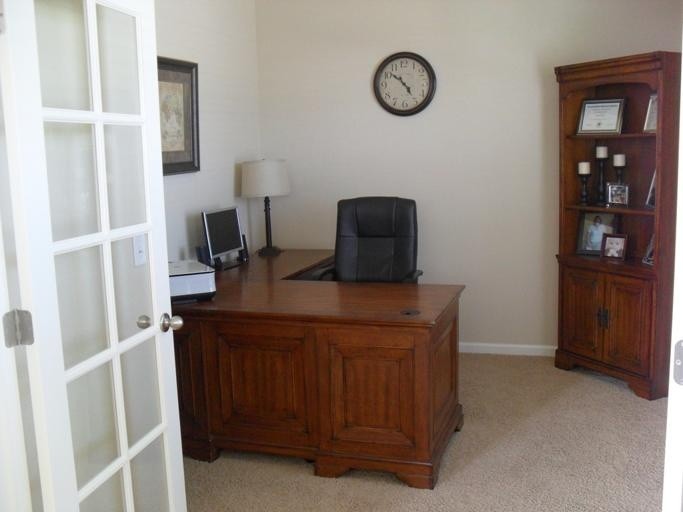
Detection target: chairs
<box><xmin>318</xmin><ymin>195</ymin><xmax>423</xmax><ymax>282</ymax></box>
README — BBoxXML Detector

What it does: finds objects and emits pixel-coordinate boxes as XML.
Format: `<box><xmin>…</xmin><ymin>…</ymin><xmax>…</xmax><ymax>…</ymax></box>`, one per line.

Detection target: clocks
<box><xmin>372</xmin><ymin>51</ymin><xmax>437</xmax><ymax>117</ymax></box>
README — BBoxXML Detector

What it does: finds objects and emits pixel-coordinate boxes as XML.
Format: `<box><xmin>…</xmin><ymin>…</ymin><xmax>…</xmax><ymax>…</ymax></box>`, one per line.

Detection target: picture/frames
<box><xmin>574</xmin><ymin>93</ymin><xmax>657</xmax><ymax>136</ymax></box>
<box><xmin>604</xmin><ymin>183</ymin><xmax>630</xmax><ymax>209</ymax></box>
<box><xmin>576</xmin><ymin>210</ymin><xmax>628</xmax><ymax>263</ymax></box>
<box><xmin>156</xmin><ymin>56</ymin><xmax>200</xmax><ymax>177</ymax></box>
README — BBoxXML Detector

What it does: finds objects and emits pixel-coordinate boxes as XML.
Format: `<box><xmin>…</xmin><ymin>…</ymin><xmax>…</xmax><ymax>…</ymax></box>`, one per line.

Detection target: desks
<box><xmin>168</xmin><ymin>247</ymin><xmax>466</xmax><ymax>489</ymax></box>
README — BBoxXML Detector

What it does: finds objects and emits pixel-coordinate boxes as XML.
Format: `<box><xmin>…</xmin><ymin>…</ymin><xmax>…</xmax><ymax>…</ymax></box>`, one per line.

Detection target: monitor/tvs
<box><xmin>201</xmin><ymin>207</ymin><xmax>245</xmax><ymax>259</ymax></box>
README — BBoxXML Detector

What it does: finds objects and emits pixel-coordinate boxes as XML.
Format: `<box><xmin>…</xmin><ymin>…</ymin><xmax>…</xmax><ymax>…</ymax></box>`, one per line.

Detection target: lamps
<box><xmin>240</xmin><ymin>157</ymin><xmax>290</xmax><ymax>257</ymax></box>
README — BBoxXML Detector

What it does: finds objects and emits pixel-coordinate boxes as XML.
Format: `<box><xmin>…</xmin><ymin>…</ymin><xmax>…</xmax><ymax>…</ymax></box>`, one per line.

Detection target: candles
<box><xmin>576</xmin><ymin>145</ymin><xmax>626</xmax><ymax>176</ymax></box>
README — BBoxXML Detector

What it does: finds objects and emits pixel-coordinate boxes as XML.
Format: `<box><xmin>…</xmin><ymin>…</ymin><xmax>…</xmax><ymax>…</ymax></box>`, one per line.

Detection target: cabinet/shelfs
<box><xmin>553</xmin><ymin>51</ymin><xmax>681</xmax><ymax>403</ymax></box>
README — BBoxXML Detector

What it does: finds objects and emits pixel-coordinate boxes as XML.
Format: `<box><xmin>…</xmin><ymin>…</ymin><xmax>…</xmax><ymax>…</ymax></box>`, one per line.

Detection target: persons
<box><xmin>584</xmin><ymin>214</ymin><xmax>608</xmax><ymax>250</ymax></box>
